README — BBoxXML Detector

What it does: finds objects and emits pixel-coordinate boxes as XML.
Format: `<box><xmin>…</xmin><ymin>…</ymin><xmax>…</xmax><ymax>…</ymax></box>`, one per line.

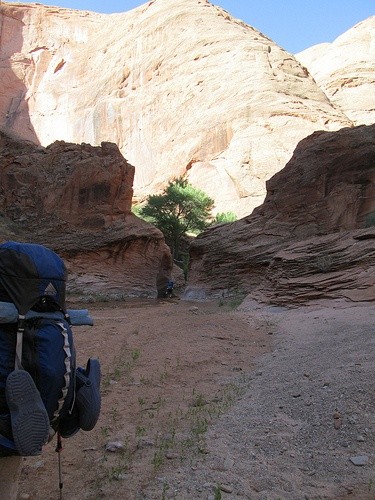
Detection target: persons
<box><xmin>166</xmin><ymin>281</ymin><xmax>174</xmax><ymax>299</ymax></box>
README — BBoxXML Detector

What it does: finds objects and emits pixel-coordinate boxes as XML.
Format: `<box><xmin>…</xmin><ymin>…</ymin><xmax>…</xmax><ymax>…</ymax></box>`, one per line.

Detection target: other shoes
<box><xmin>59</xmin><ymin>404</ymin><xmax>80</xmax><ymax>438</ymax></box>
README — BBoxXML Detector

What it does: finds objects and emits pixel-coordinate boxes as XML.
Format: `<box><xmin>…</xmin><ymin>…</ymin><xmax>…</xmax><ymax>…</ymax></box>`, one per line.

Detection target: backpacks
<box><xmin>0</xmin><ymin>240</ymin><xmax>74</xmax><ymax>452</ymax></box>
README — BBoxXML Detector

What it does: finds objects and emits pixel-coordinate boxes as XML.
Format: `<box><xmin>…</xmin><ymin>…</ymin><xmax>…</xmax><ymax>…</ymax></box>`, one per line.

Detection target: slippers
<box><xmin>75</xmin><ymin>358</ymin><xmax>101</xmax><ymax>431</ymax></box>
<box><xmin>5</xmin><ymin>370</ymin><xmax>50</xmax><ymax>457</ymax></box>
